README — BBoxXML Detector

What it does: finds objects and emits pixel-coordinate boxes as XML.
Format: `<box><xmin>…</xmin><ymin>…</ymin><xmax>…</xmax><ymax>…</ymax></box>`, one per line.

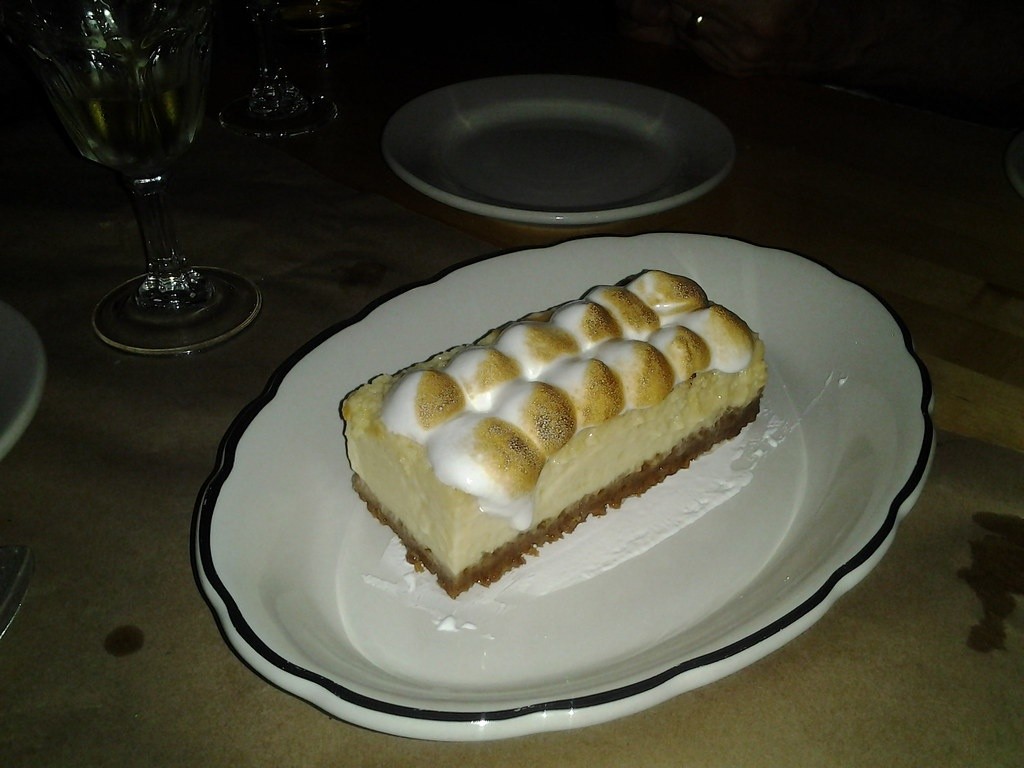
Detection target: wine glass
<box><xmin>21</xmin><ymin>0</ymin><xmax>261</xmax><ymax>353</ymax></box>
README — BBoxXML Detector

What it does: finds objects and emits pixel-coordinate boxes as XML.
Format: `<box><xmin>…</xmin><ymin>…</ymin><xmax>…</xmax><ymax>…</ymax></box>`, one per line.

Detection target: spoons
<box><xmin>2</xmin><ymin>541</ymin><xmax>32</xmax><ymax>648</ymax></box>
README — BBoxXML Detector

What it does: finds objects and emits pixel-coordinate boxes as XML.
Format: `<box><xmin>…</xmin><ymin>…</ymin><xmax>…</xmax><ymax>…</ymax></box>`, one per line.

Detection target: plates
<box><xmin>0</xmin><ymin>303</ymin><xmax>45</xmax><ymax>461</ymax></box>
<box><xmin>382</xmin><ymin>76</ymin><xmax>737</xmax><ymax>222</ymax></box>
<box><xmin>195</xmin><ymin>228</ymin><xmax>934</xmax><ymax>741</ymax></box>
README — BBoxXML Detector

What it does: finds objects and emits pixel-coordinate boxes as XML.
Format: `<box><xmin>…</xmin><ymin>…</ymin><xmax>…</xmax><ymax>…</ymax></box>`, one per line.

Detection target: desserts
<box><xmin>343</xmin><ymin>272</ymin><xmax>769</xmax><ymax>599</ymax></box>
